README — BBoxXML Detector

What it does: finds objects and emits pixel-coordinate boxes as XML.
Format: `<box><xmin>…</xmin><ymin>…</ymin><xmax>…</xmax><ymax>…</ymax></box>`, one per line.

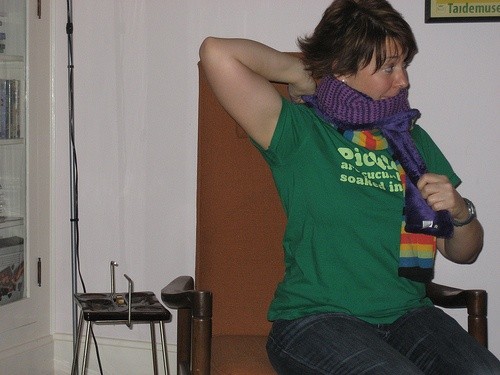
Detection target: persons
<box><xmin>198</xmin><ymin>0</ymin><xmax>500</xmax><ymax>374</ymax></box>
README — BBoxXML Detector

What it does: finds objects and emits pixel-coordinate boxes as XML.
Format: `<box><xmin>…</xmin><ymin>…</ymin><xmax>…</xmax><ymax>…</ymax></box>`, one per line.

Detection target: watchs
<box><xmin>452</xmin><ymin>197</ymin><xmax>477</xmax><ymax>227</ymax></box>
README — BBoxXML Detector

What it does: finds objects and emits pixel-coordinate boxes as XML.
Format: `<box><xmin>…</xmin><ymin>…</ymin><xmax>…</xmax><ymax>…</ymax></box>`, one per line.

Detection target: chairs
<box><xmin>160</xmin><ymin>54</ymin><xmax>488</xmax><ymax>375</ymax></box>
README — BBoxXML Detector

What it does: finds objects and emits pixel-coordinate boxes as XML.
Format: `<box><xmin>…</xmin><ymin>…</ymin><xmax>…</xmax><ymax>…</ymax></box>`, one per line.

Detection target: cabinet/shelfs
<box><xmin>0</xmin><ymin>0</ymin><xmax>51</xmax><ymax>352</ymax></box>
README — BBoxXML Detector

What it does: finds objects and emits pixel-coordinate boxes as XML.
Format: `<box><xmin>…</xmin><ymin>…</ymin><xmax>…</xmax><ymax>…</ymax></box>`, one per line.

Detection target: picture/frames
<box><xmin>424</xmin><ymin>0</ymin><xmax>500</xmax><ymax>23</ymax></box>
